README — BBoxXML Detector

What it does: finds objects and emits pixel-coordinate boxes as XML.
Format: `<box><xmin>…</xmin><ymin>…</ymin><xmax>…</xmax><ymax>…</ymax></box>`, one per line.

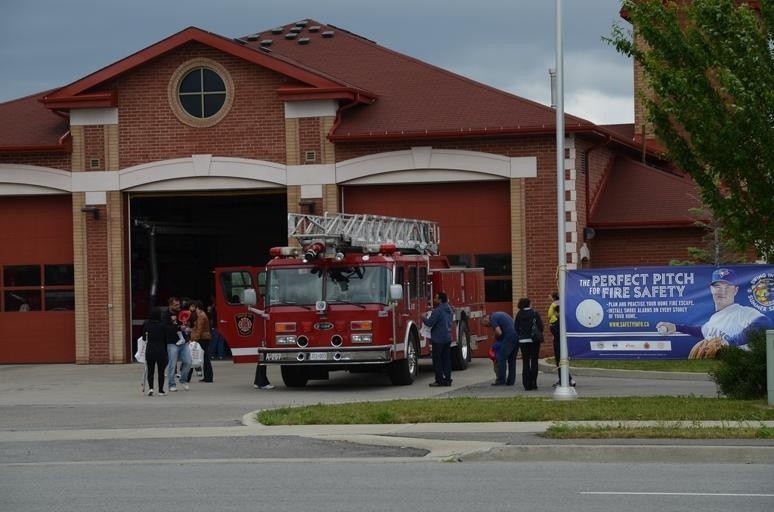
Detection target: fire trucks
<box><xmin>214</xmin><ymin>211</ymin><xmax>490</xmax><ymax>387</ymax></box>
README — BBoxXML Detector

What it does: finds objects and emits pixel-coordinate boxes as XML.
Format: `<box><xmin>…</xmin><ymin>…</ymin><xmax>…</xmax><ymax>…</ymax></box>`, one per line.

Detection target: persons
<box><xmin>480</xmin><ymin>311</ymin><xmax>519</xmax><ymax>385</ymax></box>
<box><xmin>514</xmin><ymin>298</ymin><xmax>544</xmax><ymax>391</ymax></box>
<box><xmin>421</xmin><ymin>292</ymin><xmax>453</xmax><ymax>387</ymax></box>
<box><xmin>142</xmin><ymin>293</ymin><xmax>240</xmax><ymax>395</ymax></box>
<box><xmin>549</xmin><ymin>305</ymin><xmax>577</xmax><ymax>388</ymax></box>
<box><xmin>253</xmin><ymin>362</ymin><xmax>275</xmax><ymax>389</ymax></box>
<box><xmin>656</xmin><ymin>268</ymin><xmax>774</xmax><ymax>359</ymax></box>
<box><xmin>4</xmin><ymin>279</ymin><xmax>32</xmax><ymax>311</ymax></box>
<box><xmin>547</xmin><ymin>290</ymin><xmax>558</xmax><ymax>325</ymax></box>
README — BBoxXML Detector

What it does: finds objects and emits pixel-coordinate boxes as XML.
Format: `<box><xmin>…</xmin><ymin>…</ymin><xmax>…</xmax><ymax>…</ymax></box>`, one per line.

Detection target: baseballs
<box><xmin>657</xmin><ymin>326</ymin><xmax>667</xmax><ymax>336</ymax></box>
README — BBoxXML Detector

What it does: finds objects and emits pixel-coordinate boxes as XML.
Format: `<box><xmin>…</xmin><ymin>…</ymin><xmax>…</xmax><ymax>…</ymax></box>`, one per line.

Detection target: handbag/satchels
<box><xmin>531</xmin><ymin>324</ymin><xmax>544</xmax><ymax>342</ymax></box>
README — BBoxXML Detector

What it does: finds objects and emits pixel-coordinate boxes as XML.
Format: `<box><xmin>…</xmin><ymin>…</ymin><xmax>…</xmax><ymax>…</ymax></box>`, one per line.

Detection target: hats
<box><xmin>709</xmin><ymin>269</ymin><xmax>738</xmax><ymax>286</ymax></box>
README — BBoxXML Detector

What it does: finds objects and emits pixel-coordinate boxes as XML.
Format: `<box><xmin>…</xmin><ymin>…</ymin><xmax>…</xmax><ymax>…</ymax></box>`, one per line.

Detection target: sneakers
<box><xmin>158</xmin><ymin>392</ymin><xmax>166</xmax><ymax>396</ymax></box>
<box><xmin>148</xmin><ymin>389</ymin><xmax>154</xmax><ymax>396</ymax></box>
<box><xmin>429</xmin><ymin>382</ymin><xmax>438</xmax><ymax>386</ymax></box>
<box><xmin>168</xmin><ymin>387</ymin><xmax>177</xmax><ymax>392</ymax></box>
<box><xmin>182</xmin><ymin>382</ymin><xmax>189</xmax><ymax>390</ymax></box>
<box><xmin>176</xmin><ymin>339</ymin><xmax>185</xmax><ymax>345</ymax></box>
<box><xmin>253</xmin><ymin>384</ymin><xmax>275</xmax><ymax>389</ymax></box>
